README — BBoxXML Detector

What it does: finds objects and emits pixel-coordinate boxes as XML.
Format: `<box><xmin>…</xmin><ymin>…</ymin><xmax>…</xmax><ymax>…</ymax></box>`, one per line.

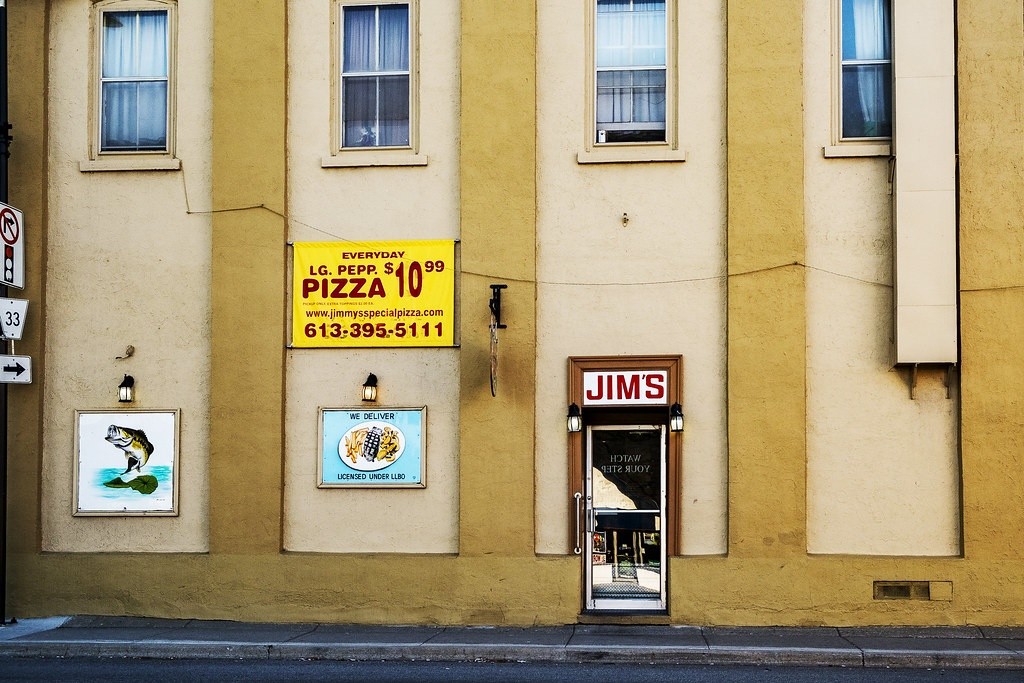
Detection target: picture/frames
<box><xmin>315</xmin><ymin>404</ymin><xmax>428</xmax><ymax>489</ymax></box>
<box><xmin>71</xmin><ymin>406</ymin><xmax>180</xmax><ymax>517</ymax></box>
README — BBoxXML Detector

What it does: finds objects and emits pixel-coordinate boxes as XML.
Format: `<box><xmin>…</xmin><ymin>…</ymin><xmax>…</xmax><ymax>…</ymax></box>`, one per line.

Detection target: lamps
<box><xmin>361</xmin><ymin>372</ymin><xmax>380</xmax><ymax>402</ymax></box>
<box><xmin>669</xmin><ymin>401</ymin><xmax>686</xmax><ymax>432</ymax></box>
<box><xmin>564</xmin><ymin>400</ymin><xmax>584</xmax><ymax>434</ymax></box>
<box><xmin>117</xmin><ymin>373</ymin><xmax>135</xmax><ymax>404</ymax></box>
